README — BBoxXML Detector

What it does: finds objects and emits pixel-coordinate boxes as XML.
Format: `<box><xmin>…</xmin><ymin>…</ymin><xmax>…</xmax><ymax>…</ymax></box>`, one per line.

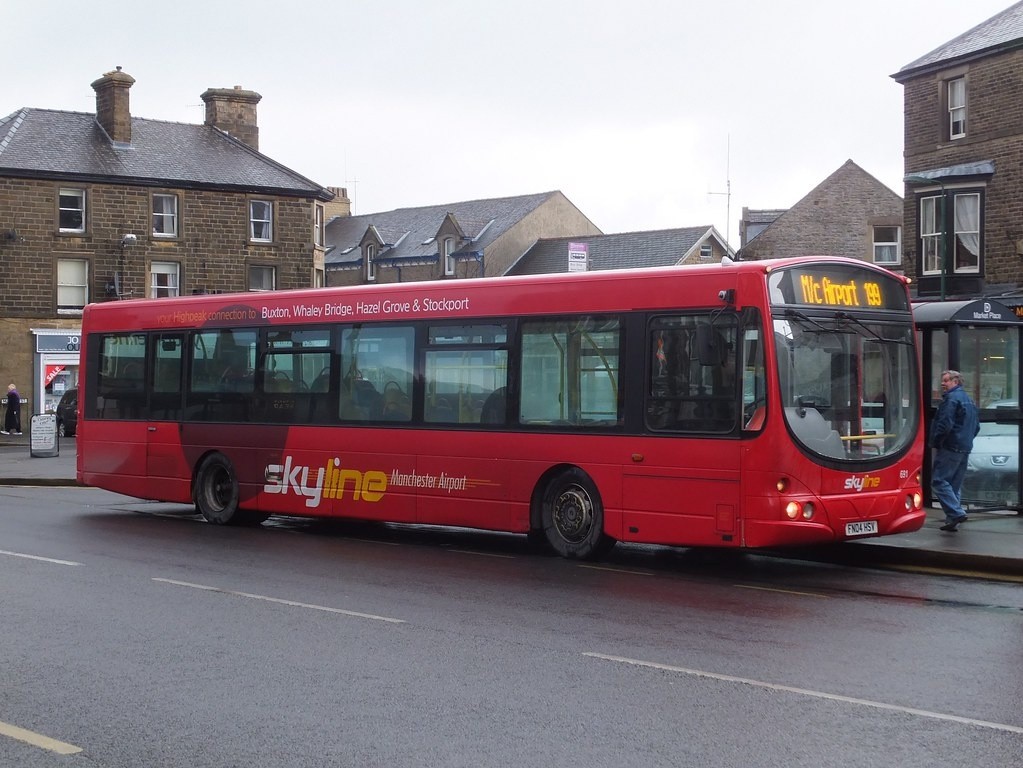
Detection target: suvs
<box><xmin>57</xmin><ymin>387</ymin><xmax>76</xmax><ymax>437</ymax></box>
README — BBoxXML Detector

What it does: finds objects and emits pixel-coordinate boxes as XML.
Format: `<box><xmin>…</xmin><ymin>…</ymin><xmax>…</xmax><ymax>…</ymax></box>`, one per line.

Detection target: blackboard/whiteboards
<box><xmin>30</xmin><ymin>414</ymin><xmax>59</xmax><ymax>449</ymax></box>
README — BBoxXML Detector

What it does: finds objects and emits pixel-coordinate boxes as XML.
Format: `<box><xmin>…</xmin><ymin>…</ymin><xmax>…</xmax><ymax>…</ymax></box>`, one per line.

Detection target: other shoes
<box><xmin>0</xmin><ymin>431</ymin><xmax>10</xmax><ymax>434</ymax></box>
<box><xmin>939</xmin><ymin>514</ymin><xmax>968</xmax><ymax>530</ymax></box>
<box><xmin>12</xmin><ymin>431</ymin><xmax>23</xmax><ymax>435</ymax></box>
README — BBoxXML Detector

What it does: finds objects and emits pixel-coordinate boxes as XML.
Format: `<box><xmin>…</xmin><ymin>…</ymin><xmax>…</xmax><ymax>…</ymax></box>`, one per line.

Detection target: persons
<box><xmin>1</xmin><ymin>384</ymin><xmax>22</xmax><ymax>435</ymax></box>
<box><xmin>929</xmin><ymin>370</ymin><xmax>981</xmax><ymax>530</ymax></box>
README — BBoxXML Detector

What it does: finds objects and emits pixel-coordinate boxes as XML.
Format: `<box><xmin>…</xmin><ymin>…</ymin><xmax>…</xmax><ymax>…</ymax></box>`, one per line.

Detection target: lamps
<box><xmin>122</xmin><ymin>233</ymin><xmax>137</xmax><ymax>247</ymax></box>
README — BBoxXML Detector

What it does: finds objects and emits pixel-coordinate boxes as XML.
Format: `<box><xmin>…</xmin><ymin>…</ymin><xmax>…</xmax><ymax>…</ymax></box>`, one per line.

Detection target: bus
<box><xmin>76</xmin><ymin>256</ymin><xmax>924</xmax><ymax>561</ymax></box>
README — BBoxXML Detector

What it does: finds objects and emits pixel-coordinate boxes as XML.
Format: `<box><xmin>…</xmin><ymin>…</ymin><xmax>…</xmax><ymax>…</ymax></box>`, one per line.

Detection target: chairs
<box><xmin>125</xmin><ymin>360</ymin><xmax>402</xmax><ymax>420</ymax></box>
